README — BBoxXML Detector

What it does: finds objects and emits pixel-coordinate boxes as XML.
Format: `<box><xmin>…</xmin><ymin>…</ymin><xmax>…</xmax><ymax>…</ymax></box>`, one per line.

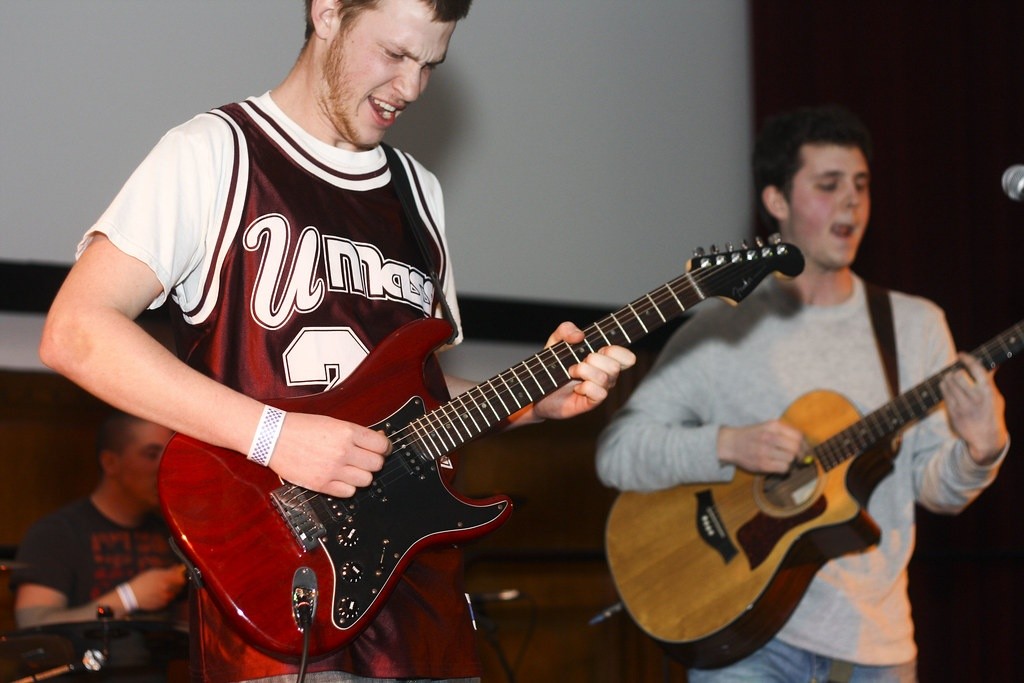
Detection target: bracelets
<box><xmin>245</xmin><ymin>405</ymin><xmax>288</xmax><ymax>468</ymax></box>
<box><xmin>116</xmin><ymin>582</ymin><xmax>140</xmax><ymax>616</ymax></box>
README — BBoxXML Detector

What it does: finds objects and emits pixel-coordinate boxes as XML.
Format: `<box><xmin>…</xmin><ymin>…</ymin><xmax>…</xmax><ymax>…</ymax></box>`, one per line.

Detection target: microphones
<box><xmin>590</xmin><ymin>603</ymin><xmax>622</xmax><ymax>626</ymax></box>
<box><xmin>476</xmin><ymin>589</ymin><xmax>526</xmax><ymax>602</ymax></box>
<box><xmin>1002</xmin><ymin>164</ymin><xmax>1024</xmax><ymax>200</ymax></box>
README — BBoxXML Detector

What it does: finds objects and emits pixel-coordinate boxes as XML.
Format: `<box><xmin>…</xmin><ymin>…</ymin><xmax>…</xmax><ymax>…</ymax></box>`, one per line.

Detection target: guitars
<box><xmin>156</xmin><ymin>231</ymin><xmax>807</xmax><ymax>665</ymax></box>
<box><xmin>600</xmin><ymin>317</ymin><xmax>1024</xmax><ymax>670</ymax></box>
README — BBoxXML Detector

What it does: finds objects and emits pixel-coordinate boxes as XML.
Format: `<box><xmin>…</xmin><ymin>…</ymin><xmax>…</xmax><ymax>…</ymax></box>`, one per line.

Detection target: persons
<box><xmin>39</xmin><ymin>0</ymin><xmax>639</xmax><ymax>683</ymax></box>
<box><xmin>596</xmin><ymin>112</ymin><xmax>1013</xmax><ymax>683</ymax></box>
<box><xmin>13</xmin><ymin>414</ymin><xmax>204</xmax><ymax>629</ymax></box>
